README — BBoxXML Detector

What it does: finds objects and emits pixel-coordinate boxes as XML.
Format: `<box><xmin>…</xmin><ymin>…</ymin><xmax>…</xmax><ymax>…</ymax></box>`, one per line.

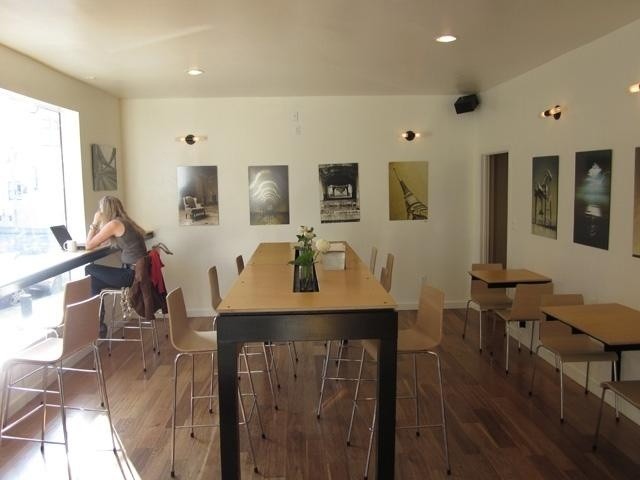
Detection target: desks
<box><xmin>215</xmin><ymin>241</ymin><xmax>399</xmax><ymax>480</ymax></box>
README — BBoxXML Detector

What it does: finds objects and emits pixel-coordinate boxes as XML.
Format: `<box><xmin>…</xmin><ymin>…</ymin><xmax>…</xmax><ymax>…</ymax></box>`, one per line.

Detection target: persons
<box><xmin>84</xmin><ymin>194</ymin><xmax>149</xmax><ymax>338</ymax></box>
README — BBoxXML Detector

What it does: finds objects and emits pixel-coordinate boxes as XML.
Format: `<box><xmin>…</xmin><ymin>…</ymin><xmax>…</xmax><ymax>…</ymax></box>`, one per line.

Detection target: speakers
<box><xmin>453</xmin><ymin>94</ymin><xmax>479</xmax><ymax>115</ymax></box>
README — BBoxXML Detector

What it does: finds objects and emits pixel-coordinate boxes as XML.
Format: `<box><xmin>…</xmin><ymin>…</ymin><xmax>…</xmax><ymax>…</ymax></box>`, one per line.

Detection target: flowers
<box><xmin>286</xmin><ymin>239</ymin><xmax>332</xmax><ymax>289</ymax></box>
<box><xmin>294</xmin><ymin>225</ymin><xmax>317</xmax><ymax>254</ymax></box>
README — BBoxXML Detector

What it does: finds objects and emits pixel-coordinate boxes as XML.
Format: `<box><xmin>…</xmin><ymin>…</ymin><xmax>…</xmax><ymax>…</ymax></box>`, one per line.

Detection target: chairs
<box><xmin>208</xmin><ymin>266</ymin><xmax>280</xmax><ymax>414</ymax></box>
<box><xmin>317</xmin><ymin>267</ymin><xmax>391</xmax><ymax>419</ymax></box>
<box><xmin>236</xmin><ymin>255</ymin><xmax>299</xmax><ymax>380</ymax></box>
<box><xmin>463</xmin><ymin>263</ymin><xmax>640</xmax><ymax>454</ymax></box>
<box><xmin>165</xmin><ymin>286</ymin><xmax>266</xmax><ymax>477</ymax></box>
<box><xmin>324</xmin><ymin>246</ymin><xmax>377</xmax><ymax>347</ymax></box>
<box><xmin>346</xmin><ymin>284</ymin><xmax>451</xmax><ymax>480</ymax></box>
<box><xmin>335</xmin><ymin>253</ymin><xmax>394</xmax><ymax>381</ymax></box>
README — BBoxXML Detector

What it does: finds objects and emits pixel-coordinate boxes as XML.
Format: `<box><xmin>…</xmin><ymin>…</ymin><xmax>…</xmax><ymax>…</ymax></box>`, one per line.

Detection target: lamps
<box><xmin>400</xmin><ymin>130</ymin><xmax>421</xmax><ymax>143</ymax></box>
<box><xmin>627</xmin><ymin>80</ymin><xmax>640</xmax><ymax>94</ymax></box>
<box><xmin>176</xmin><ymin>133</ymin><xmax>201</xmax><ymax>146</ymax></box>
<box><xmin>540</xmin><ymin>105</ymin><xmax>562</xmax><ymax>120</ymax></box>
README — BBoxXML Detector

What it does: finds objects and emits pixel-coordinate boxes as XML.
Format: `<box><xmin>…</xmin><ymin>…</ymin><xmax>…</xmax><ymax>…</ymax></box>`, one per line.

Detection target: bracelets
<box><xmin>89</xmin><ymin>224</ymin><xmax>98</xmax><ymax>231</ymax></box>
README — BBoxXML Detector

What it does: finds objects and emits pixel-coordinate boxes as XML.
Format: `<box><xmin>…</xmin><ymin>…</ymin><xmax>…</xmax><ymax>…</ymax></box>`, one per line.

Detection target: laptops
<box><xmin>49</xmin><ymin>225</ymin><xmax>85</xmax><ymax>250</ymax></box>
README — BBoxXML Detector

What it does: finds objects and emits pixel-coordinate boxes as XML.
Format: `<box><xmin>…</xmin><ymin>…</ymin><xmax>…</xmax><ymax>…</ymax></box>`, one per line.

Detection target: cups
<box><xmin>63</xmin><ymin>240</ymin><xmax>77</xmax><ymax>252</ymax></box>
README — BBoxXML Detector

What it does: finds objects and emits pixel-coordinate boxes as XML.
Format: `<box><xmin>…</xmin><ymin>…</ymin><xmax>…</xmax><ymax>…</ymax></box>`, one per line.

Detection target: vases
<box><xmin>298</xmin><ymin>251</ymin><xmax>313</xmax><ymax>292</ymax></box>
<box><xmin>299</xmin><ymin>250</ymin><xmax>304</xmax><ymax>256</ymax></box>
